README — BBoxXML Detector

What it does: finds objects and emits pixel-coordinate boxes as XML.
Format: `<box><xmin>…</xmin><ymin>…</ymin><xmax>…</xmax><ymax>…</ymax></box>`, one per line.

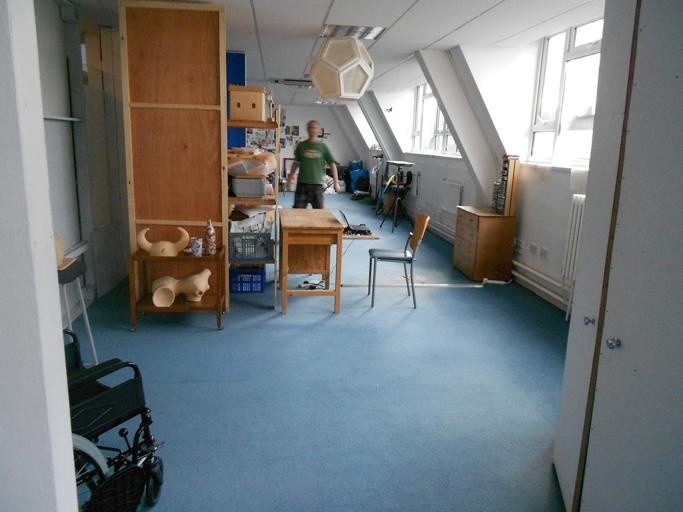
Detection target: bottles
<box><xmin>204</xmin><ymin>219</ymin><xmax>217</xmax><ymax>255</ymax></box>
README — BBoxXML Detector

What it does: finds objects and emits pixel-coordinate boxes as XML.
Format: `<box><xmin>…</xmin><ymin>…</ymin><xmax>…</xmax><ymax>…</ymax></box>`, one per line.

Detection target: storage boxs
<box><xmin>228</xmin><ymin>85</ymin><xmax>272</xmax><ymax>122</ymax></box>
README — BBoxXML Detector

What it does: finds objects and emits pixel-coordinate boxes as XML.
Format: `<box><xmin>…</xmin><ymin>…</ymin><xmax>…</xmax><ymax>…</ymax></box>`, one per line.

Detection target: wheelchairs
<box><xmin>62</xmin><ymin>328</ymin><xmax>167</xmax><ymax>511</ymax></box>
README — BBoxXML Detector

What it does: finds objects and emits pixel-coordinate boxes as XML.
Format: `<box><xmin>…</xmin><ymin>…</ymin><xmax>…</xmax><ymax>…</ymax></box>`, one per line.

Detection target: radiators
<box><xmin>440</xmin><ymin>180</ymin><xmax>462</xmax><ymax>216</ymax></box>
<box><xmin>562</xmin><ymin>194</ymin><xmax>586</xmax><ymax>282</ymax></box>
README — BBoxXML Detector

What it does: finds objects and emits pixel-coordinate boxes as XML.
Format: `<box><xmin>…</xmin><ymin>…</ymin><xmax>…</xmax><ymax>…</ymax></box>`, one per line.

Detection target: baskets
<box><xmin>229</xmin><ymin>264</ymin><xmax>265</xmax><ymax>294</ymax></box>
<box><xmin>228</xmin><ymin>232</ymin><xmax>273</xmax><ymax>261</ymax></box>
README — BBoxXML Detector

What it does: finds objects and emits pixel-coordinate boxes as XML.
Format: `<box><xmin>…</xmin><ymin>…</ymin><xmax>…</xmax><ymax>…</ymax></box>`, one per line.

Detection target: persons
<box><xmin>287</xmin><ymin>121</ymin><xmax>341</xmax><ymax>209</ymax></box>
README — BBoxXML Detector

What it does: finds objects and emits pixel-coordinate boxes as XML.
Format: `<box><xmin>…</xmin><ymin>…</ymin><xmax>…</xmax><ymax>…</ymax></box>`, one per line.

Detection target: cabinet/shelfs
<box><xmin>453</xmin><ymin>206</ymin><xmax>518</xmax><ymax>282</ymax></box>
<box><xmin>227</xmin><ymin>105</ymin><xmax>280</xmax><ymax>311</ymax></box>
<box><xmin>128</xmin><ymin>246</ymin><xmax>226</xmax><ymax>331</ymax></box>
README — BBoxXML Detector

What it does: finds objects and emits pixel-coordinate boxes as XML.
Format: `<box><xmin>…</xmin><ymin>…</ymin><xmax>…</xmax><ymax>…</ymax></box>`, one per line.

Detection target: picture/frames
<box><xmin>284</xmin><ymin>158</ymin><xmax>301</xmax><ymax>178</ymax></box>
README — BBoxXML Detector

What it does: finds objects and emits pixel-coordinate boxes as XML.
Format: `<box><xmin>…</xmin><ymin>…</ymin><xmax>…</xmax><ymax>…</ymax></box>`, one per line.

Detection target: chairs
<box><xmin>368</xmin><ymin>214</ymin><xmax>431</xmax><ymax>309</ymax></box>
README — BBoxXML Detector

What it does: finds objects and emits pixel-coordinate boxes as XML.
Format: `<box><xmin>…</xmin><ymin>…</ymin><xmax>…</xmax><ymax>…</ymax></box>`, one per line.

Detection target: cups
<box><xmin>190</xmin><ymin>237</ymin><xmax>203</xmax><ymax>257</ymax></box>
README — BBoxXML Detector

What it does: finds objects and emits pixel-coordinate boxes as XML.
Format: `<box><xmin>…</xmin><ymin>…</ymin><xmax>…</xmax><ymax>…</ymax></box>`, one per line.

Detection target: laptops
<box><xmin>338</xmin><ymin>210</ymin><xmax>370</xmax><ymax>234</ymax></box>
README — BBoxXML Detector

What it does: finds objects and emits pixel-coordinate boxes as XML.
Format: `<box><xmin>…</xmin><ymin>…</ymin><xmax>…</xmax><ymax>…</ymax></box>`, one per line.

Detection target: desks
<box><xmin>278</xmin><ymin>208</ymin><xmax>345</xmax><ymax>315</ymax></box>
